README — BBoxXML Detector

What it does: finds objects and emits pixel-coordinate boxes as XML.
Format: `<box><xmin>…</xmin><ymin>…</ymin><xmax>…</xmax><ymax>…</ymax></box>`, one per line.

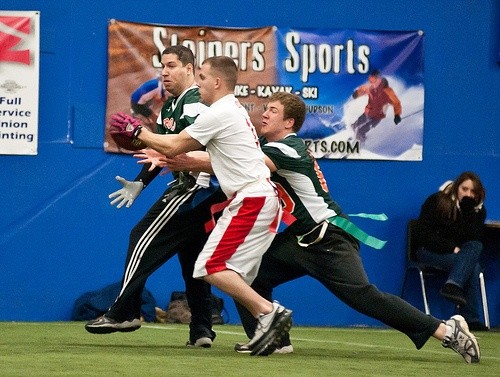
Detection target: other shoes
<box><xmin>438</xmin><ymin>286</ymin><xmax>466</xmax><ymax>306</ymax></box>
<box><xmin>467</xmin><ymin>321</ymin><xmax>489</xmax><ymax>332</ymax></box>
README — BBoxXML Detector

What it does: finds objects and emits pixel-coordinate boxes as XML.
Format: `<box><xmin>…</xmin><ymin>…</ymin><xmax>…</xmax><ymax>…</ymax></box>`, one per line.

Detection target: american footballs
<box><xmin>107</xmin><ymin>114</ymin><xmax>153</xmax><ymax>151</ymax></box>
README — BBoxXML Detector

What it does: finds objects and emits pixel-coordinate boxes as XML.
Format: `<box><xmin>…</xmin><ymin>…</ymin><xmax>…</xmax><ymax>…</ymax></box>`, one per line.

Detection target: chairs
<box><xmin>400</xmin><ymin>219</ymin><xmax>490</xmax><ymax>331</ymax></box>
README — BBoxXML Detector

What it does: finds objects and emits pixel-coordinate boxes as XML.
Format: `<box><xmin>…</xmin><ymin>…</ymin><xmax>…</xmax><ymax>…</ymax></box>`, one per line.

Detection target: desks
<box><xmin>485</xmin><ymin>220</ymin><xmax>500</xmax><ymax>228</ymax></box>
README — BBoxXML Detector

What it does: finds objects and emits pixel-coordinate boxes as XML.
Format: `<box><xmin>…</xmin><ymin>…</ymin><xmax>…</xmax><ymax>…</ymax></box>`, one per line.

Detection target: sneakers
<box><xmin>249</xmin><ymin>300</ymin><xmax>293</xmax><ymax>357</ymax></box>
<box><xmin>186</xmin><ymin>337</ymin><xmax>213</xmax><ymax>348</ymax></box>
<box><xmin>84</xmin><ymin>314</ymin><xmax>141</xmax><ymax>335</ymax></box>
<box><xmin>234</xmin><ymin>331</ymin><xmax>294</xmax><ymax>354</ymax></box>
<box><xmin>440</xmin><ymin>315</ymin><xmax>481</xmax><ymax>364</ymax></box>
<box><xmin>249</xmin><ymin>314</ymin><xmax>293</xmax><ymax>356</ymax></box>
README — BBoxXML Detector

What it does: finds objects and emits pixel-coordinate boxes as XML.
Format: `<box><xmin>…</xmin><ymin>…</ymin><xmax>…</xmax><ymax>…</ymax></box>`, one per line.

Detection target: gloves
<box><xmin>109</xmin><ymin>112</ymin><xmax>144</xmax><ymax>141</ymax></box>
<box><xmin>459</xmin><ymin>196</ymin><xmax>475</xmax><ymax>218</ymax></box>
<box><xmin>394</xmin><ymin>114</ymin><xmax>401</xmax><ymax>124</ymax></box>
<box><xmin>352</xmin><ymin>90</ymin><xmax>358</xmax><ymax>98</ymax></box>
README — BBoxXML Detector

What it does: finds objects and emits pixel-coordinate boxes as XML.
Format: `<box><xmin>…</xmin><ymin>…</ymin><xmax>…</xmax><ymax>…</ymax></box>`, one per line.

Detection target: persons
<box><xmin>130</xmin><ymin>78</ymin><xmax>168</xmax><ymax>132</ymax></box>
<box><xmin>349</xmin><ymin>69</ymin><xmax>401</xmax><ymax>147</ymax></box>
<box><xmin>108</xmin><ymin>55</ymin><xmax>293</xmax><ymax>357</ymax></box>
<box><xmin>133</xmin><ymin>91</ymin><xmax>481</xmax><ymax>363</ymax></box>
<box><xmin>414</xmin><ymin>171</ymin><xmax>489</xmax><ymax>329</ymax></box>
<box><xmin>85</xmin><ymin>44</ymin><xmax>228</xmax><ymax>349</ymax></box>
